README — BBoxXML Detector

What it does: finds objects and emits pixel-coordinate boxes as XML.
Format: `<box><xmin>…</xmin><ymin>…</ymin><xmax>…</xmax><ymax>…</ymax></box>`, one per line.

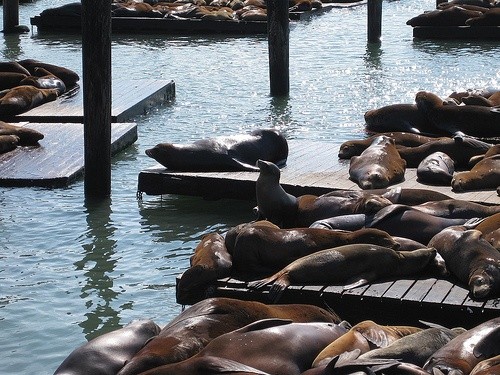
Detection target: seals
<box><xmin>144</xmin><ymin>126</ymin><xmax>289</xmax><ymax>172</ymax></box>
<box><xmin>406</xmin><ymin>0</ymin><xmax>500</xmax><ymax>26</ymax></box>
<box><xmin>52</xmin><ymin>159</ymin><xmax>500</xmax><ymax>375</ymax></box>
<box><xmin>0</xmin><ymin>58</ymin><xmax>80</xmax><ymax>151</ymax></box>
<box><xmin>39</xmin><ymin>0</ymin><xmax>323</xmax><ymax>23</ymax></box>
<box><xmin>337</xmin><ymin>85</ymin><xmax>500</xmax><ymax>196</ymax></box>
